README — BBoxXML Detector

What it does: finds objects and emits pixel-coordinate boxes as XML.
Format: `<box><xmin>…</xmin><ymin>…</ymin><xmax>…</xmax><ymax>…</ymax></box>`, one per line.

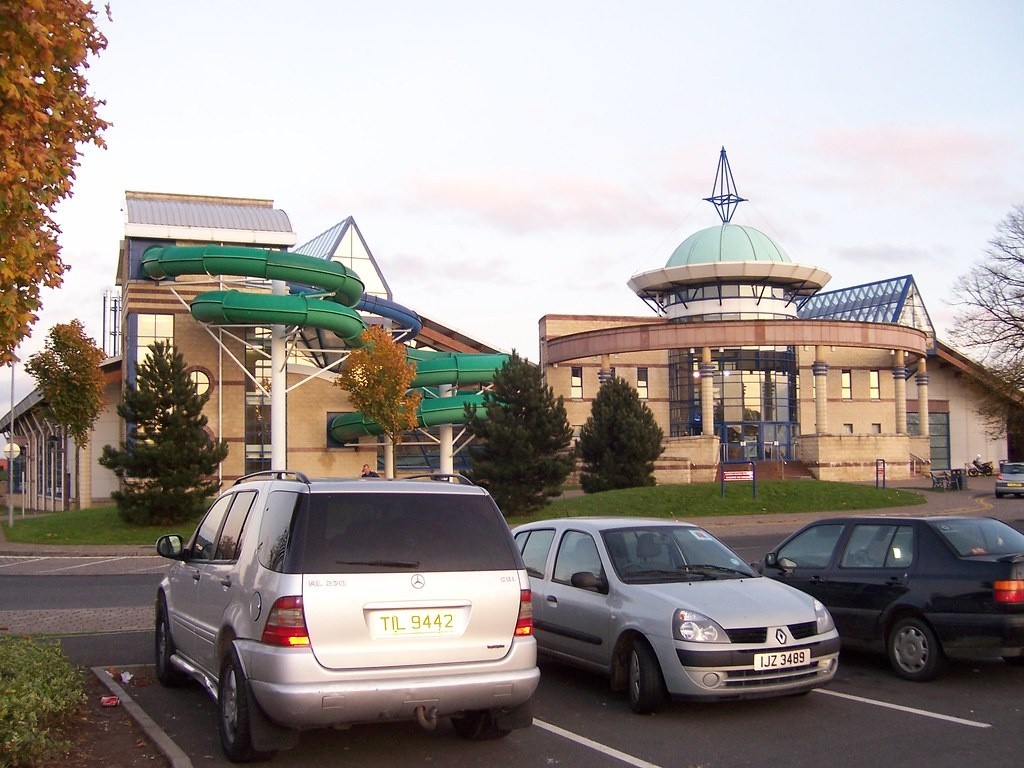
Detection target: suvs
<box><xmin>154</xmin><ymin>471</ymin><xmax>540</xmax><ymax>764</ymax></box>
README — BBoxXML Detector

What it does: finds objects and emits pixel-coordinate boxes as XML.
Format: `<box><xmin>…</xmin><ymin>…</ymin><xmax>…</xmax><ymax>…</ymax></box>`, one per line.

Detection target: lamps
<box><xmin>689</xmin><ymin>348</ymin><xmax>696</xmax><ymax>354</ymax></box>
<box><xmin>615</xmin><ymin>353</ymin><xmax>619</xmax><ymax>359</ymax></box>
<box><xmin>804</xmin><ymin>346</ymin><xmax>809</xmax><ymax>352</ymax></box>
<box><xmin>718</xmin><ymin>347</ymin><xmax>725</xmax><ymax>353</ymax></box>
<box><xmin>904</xmin><ymin>351</ymin><xmax>909</xmax><ymax>356</ymax></box>
<box><xmin>830</xmin><ymin>346</ymin><xmax>835</xmax><ymax>352</ymax></box>
<box><xmin>552</xmin><ymin>362</ymin><xmax>559</xmax><ymax>368</ymax></box>
<box><xmin>890</xmin><ymin>349</ymin><xmax>895</xmax><ymax>356</ymax></box>
<box><xmin>593</xmin><ymin>356</ymin><xmax>597</xmax><ymax>361</ymax></box>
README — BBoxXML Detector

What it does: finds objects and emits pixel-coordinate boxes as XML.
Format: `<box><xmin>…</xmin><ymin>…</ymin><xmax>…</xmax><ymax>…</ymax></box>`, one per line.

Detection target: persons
<box><xmin>358</xmin><ymin>464</ymin><xmax>379</xmax><ymax>478</ymax></box>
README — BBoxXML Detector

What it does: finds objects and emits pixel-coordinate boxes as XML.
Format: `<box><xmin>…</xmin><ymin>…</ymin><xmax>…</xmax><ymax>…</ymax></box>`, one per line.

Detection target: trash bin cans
<box><xmin>951</xmin><ymin>468</ymin><xmax>968</xmax><ymax>490</ymax></box>
<box><xmin>1000</xmin><ymin>460</ymin><xmax>1009</xmax><ymax>473</ymax></box>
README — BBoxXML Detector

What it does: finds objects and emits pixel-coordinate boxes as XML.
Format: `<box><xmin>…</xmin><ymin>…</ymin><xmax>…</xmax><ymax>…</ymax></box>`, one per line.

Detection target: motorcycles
<box><xmin>967</xmin><ymin>455</ymin><xmax>993</xmax><ymax>476</ymax></box>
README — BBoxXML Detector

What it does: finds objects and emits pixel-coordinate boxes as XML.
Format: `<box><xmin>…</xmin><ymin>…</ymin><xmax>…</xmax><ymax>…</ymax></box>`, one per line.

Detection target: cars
<box><xmin>504</xmin><ymin>518</ymin><xmax>840</xmax><ymax>714</ymax></box>
<box><xmin>748</xmin><ymin>514</ymin><xmax>1024</xmax><ymax>682</ymax></box>
<box><xmin>995</xmin><ymin>464</ymin><xmax>1024</xmax><ymax>498</ymax></box>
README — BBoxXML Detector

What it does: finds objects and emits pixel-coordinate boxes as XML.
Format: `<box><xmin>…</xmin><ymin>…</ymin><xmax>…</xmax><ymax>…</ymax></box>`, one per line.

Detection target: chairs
<box><xmin>857</xmin><ymin>541</ymin><xmax>891</xmax><ymax>568</ymax></box>
<box><xmin>929</xmin><ymin>472</ymin><xmax>960</xmax><ymax>491</ymax></box>
<box><xmin>627</xmin><ymin>533</ymin><xmax>670</xmax><ymax>573</ymax></box>
